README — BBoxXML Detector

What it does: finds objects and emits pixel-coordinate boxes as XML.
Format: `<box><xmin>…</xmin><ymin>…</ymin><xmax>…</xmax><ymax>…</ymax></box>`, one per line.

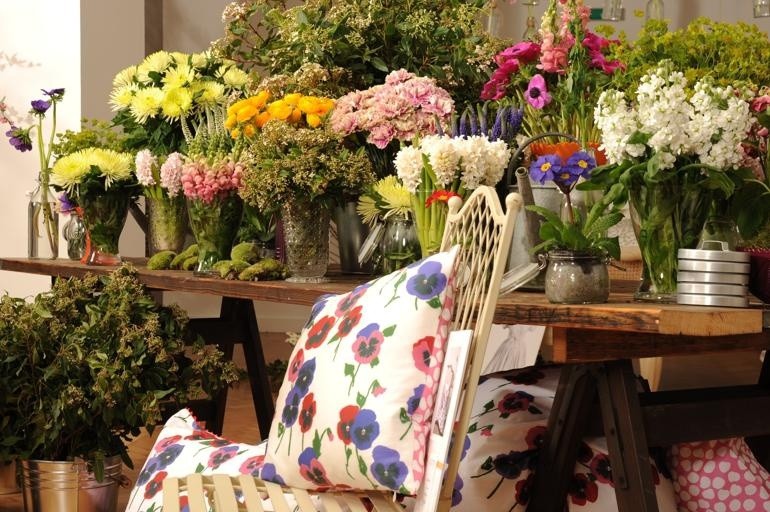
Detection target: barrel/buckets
<box><xmin>75</xmin><ymin>454</ymin><xmax>132</xmax><ymax>512</ymax></box>
<box><xmin>332</xmin><ymin>198</ymin><xmax>372</xmax><ymax>275</ymax></box>
<box><xmin>22</xmin><ymin>460</ymin><xmax>78</xmax><ymax>511</ymax></box>
<box><xmin>497</xmin><ymin>130</ymin><xmax>577</xmax><ymax>289</ymax></box>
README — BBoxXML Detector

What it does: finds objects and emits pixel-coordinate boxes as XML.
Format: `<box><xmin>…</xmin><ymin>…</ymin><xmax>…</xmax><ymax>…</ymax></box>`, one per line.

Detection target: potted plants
<box><xmin>0</xmin><ymin>264</ymin><xmax>238</xmax><ymax>512</ymax></box>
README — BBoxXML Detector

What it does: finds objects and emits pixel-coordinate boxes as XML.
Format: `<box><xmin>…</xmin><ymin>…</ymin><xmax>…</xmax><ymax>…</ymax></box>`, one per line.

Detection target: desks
<box><xmin>0</xmin><ymin>258</ymin><xmax>769</xmax><ymax>512</ymax></box>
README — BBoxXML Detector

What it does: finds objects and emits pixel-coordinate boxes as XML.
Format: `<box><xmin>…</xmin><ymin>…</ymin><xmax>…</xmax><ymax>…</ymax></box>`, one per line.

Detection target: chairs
<box><xmin>162</xmin><ymin>185</ymin><xmax>523</xmax><ymax>512</ymax></box>
